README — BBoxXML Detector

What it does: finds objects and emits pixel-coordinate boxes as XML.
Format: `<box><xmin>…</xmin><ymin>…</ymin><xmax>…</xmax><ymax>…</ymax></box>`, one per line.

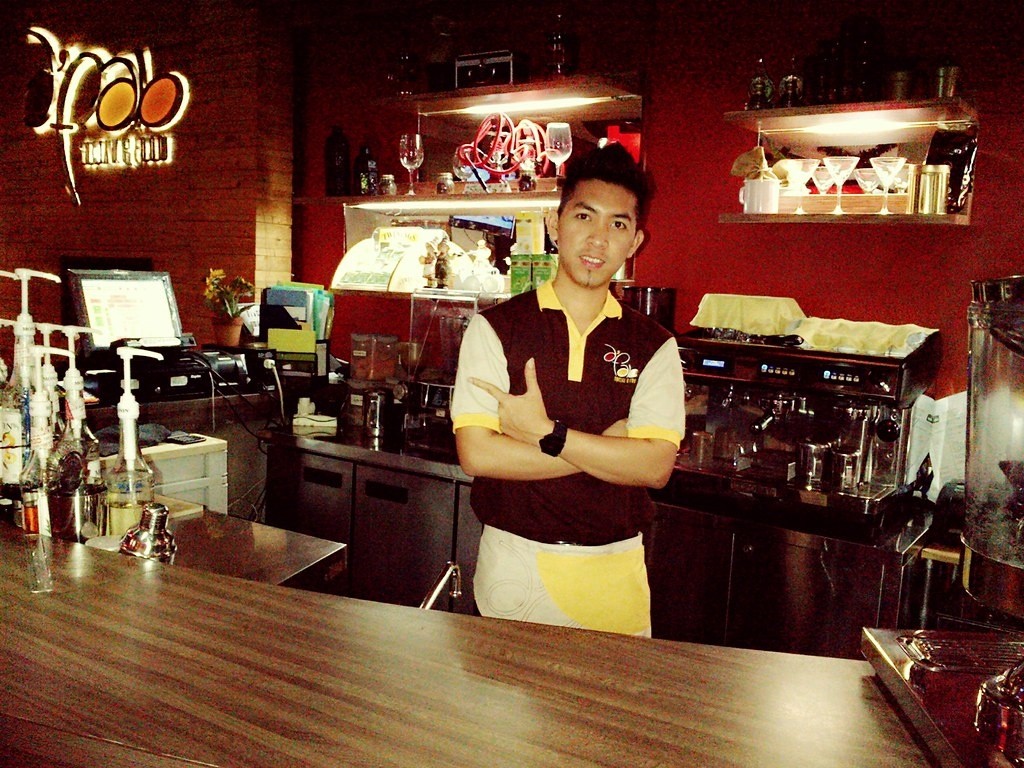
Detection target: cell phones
<box><xmin>167</xmin><ymin>435</ymin><xmax>206</xmax><ymax>445</ymax></box>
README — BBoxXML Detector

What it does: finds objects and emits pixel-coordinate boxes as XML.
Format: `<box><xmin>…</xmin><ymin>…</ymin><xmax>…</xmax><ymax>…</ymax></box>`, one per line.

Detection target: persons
<box><xmin>450</xmin><ymin>141</ymin><xmax>686</xmax><ymax>639</ymax></box>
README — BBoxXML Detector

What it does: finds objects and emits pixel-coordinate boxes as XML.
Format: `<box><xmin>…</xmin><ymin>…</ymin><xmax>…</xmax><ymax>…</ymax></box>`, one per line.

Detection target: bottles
<box><xmin>907</xmin><ymin>164</ymin><xmax>950</xmax><ymax>214</ymax></box>
<box><xmin>353</xmin><ymin>146</ymin><xmax>396</xmax><ymax>194</ymax></box>
<box><xmin>324</xmin><ymin>126</ymin><xmax>349</xmax><ymax>196</ymax></box>
<box><xmin>437</xmin><ymin>172</ymin><xmax>454</xmax><ymax>194</ymax></box>
<box><xmin>118</xmin><ymin>501</ymin><xmax>178</xmax><ymax>565</ymax></box>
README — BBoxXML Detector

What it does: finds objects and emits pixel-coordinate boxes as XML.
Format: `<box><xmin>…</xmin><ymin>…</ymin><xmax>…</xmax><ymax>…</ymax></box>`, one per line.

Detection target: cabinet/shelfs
<box><xmin>717</xmin><ymin>97</ymin><xmax>981</xmax><ymax>225</ymax></box>
<box><xmin>644</xmin><ymin>516</ymin><xmax>946</xmax><ymax>660</ymax></box>
<box><xmin>322</xmin><ymin>76</ymin><xmax>646</xmax><ymax>307</ymax></box>
<box><xmin>264</xmin><ymin>444</ymin><xmax>483</xmax><ymax>616</ymax></box>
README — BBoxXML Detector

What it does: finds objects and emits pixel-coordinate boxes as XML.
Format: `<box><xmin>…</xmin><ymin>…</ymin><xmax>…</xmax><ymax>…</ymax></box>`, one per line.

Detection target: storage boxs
<box><xmin>511</xmin><ymin>254</ymin><xmax>559</xmax><ymax>295</ymax></box>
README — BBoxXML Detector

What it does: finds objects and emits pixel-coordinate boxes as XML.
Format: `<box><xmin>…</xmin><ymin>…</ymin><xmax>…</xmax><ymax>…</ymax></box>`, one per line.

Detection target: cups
<box><xmin>622</xmin><ymin>286</ymin><xmax>675</xmax><ymax>334</ymax></box>
<box><xmin>452</xmin><ymin>146</ymin><xmax>475</xmax><ymax>181</ymax></box>
<box><xmin>690</xmin><ymin>431</ymin><xmax>713</xmax><ymax>469</ymax></box>
<box><xmin>853</xmin><ymin>168</ymin><xmax>880</xmax><ymax>194</ymax></box>
<box><xmin>345</xmin><ymin>333</ymin><xmax>398</xmax><ymax>427</ymax></box>
<box><xmin>364</xmin><ymin>389</ymin><xmax>386</xmax><ymax>437</ymax></box>
<box><xmin>738</xmin><ymin>179</ymin><xmax>780</xmax><ymax>214</ymax></box>
<box><xmin>48</xmin><ymin>484</ymin><xmax>110</xmax><ymax>544</ymax></box>
<box><xmin>797</xmin><ymin>432</ymin><xmax>861</xmax><ymax>491</ymax></box>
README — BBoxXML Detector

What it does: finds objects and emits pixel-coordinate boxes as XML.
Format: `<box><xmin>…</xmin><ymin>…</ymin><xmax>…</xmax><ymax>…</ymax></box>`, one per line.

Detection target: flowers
<box><xmin>197</xmin><ymin>267</ymin><xmax>256</xmax><ymax>318</ymax></box>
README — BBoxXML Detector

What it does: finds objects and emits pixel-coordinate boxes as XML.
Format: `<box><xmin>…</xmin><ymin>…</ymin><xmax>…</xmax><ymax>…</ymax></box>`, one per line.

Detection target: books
<box><xmin>267</xmin><ymin>280</ymin><xmax>333</xmax><ymax>340</ymax></box>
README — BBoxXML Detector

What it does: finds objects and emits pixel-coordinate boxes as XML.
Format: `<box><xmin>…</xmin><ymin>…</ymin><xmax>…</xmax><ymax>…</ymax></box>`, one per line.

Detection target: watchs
<box><xmin>539</xmin><ymin>421</ymin><xmax>569</xmax><ymax>456</ymax></box>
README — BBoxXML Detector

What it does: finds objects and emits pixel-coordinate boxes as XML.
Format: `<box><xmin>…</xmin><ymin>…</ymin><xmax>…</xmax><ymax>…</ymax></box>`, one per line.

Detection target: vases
<box><xmin>211</xmin><ymin>316</ymin><xmax>243</xmax><ymax>346</ymax></box>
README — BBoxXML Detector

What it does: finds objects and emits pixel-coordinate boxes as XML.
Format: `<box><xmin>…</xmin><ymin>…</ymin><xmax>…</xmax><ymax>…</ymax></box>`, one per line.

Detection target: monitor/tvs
<box><xmin>64</xmin><ymin>266</ymin><xmax>183</xmax><ymax>359</ymax></box>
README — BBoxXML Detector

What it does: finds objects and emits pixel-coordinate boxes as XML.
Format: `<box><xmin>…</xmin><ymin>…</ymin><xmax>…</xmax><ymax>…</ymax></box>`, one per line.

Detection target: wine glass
<box><xmin>783</xmin><ymin>159</ymin><xmax>820</xmax><ymax>214</ymax></box>
<box><xmin>402</xmin><ymin>341</ymin><xmax>425</xmax><ymax>383</ymax></box>
<box><xmin>400</xmin><ymin>133</ymin><xmax>423</xmax><ymax>195</ymax></box>
<box><xmin>822</xmin><ymin>156</ymin><xmax>860</xmax><ymax>214</ymax></box>
<box><xmin>811</xmin><ymin>166</ymin><xmax>834</xmax><ymax>194</ymax></box>
<box><xmin>544</xmin><ymin>123</ymin><xmax>572</xmax><ymax>192</ymax></box>
<box><xmin>869</xmin><ymin>156</ymin><xmax>915</xmax><ymax>214</ymax></box>
